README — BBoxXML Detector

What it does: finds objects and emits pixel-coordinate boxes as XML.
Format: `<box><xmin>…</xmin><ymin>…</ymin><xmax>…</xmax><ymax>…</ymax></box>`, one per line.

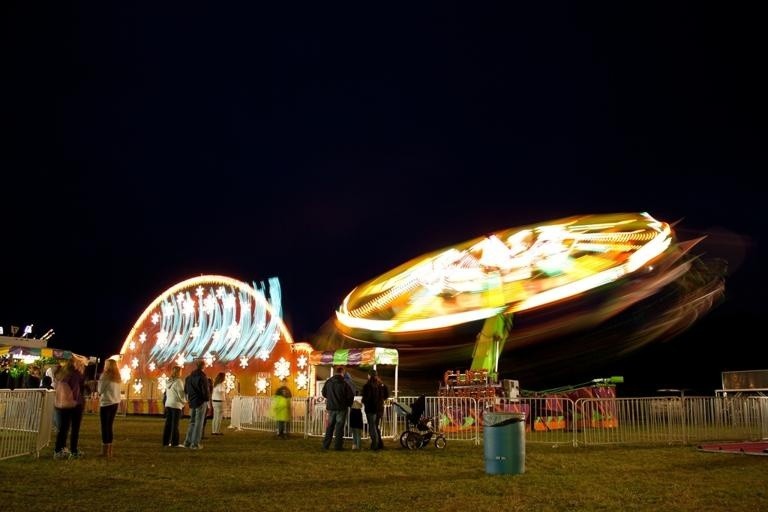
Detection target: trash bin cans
<box><xmin>483</xmin><ymin>412</ymin><xmax>526</xmax><ymax>475</ymax></box>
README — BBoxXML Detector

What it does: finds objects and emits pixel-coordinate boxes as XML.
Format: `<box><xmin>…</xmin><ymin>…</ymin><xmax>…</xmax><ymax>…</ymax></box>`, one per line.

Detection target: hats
<box><xmin>53</xmin><ymin>349</ymin><xmax>73</xmax><ymax>360</ymax></box>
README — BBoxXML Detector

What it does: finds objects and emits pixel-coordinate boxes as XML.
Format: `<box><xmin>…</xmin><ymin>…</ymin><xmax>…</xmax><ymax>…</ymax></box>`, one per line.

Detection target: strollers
<box><xmin>387</xmin><ymin>398</ymin><xmax>447</xmax><ymax>451</ymax></box>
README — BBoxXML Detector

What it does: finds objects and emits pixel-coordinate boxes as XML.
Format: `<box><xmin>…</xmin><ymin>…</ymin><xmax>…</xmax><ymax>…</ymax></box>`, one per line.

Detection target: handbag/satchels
<box><xmin>54</xmin><ymin>383</ymin><xmax>74</xmax><ymax>409</ymax></box>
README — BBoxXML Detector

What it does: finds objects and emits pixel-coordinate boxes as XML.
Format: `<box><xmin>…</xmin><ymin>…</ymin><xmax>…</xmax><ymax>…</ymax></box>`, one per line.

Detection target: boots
<box><xmin>96</xmin><ymin>442</ymin><xmax>114</xmax><ymax>458</ymax></box>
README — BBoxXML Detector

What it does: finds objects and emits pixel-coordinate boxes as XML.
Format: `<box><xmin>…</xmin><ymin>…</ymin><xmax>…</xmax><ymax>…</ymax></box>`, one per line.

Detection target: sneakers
<box><xmin>53</xmin><ymin>448</ymin><xmax>85</xmax><ymax>463</ymax></box>
<box><xmin>163</xmin><ymin>432</ymin><xmax>224</xmax><ymax>450</ymax></box>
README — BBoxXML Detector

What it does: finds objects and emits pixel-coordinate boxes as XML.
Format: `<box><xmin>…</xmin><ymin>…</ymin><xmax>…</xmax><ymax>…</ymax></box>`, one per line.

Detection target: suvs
<box><xmin>649</xmin><ymin>389</ymin><xmax>691</xmax><ymax>420</ymax></box>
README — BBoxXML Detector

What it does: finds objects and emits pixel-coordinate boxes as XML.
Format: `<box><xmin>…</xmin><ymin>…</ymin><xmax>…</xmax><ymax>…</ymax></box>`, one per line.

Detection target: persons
<box><xmin>321</xmin><ymin>367</ymin><xmax>389</xmax><ymax>450</ymax></box>
<box><xmin>271</xmin><ymin>377</ymin><xmax>292</xmax><ymax>439</ymax></box>
<box><xmin>161</xmin><ymin>359</ymin><xmax>226</xmax><ymax>451</ymax></box>
<box><xmin>0</xmin><ymin>352</ymin><xmax>86</xmax><ymax>459</ymax></box>
<box><xmin>97</xmin><ymin>358</ymin><xmax>123</xmax><ymax>459</ymax></box>
